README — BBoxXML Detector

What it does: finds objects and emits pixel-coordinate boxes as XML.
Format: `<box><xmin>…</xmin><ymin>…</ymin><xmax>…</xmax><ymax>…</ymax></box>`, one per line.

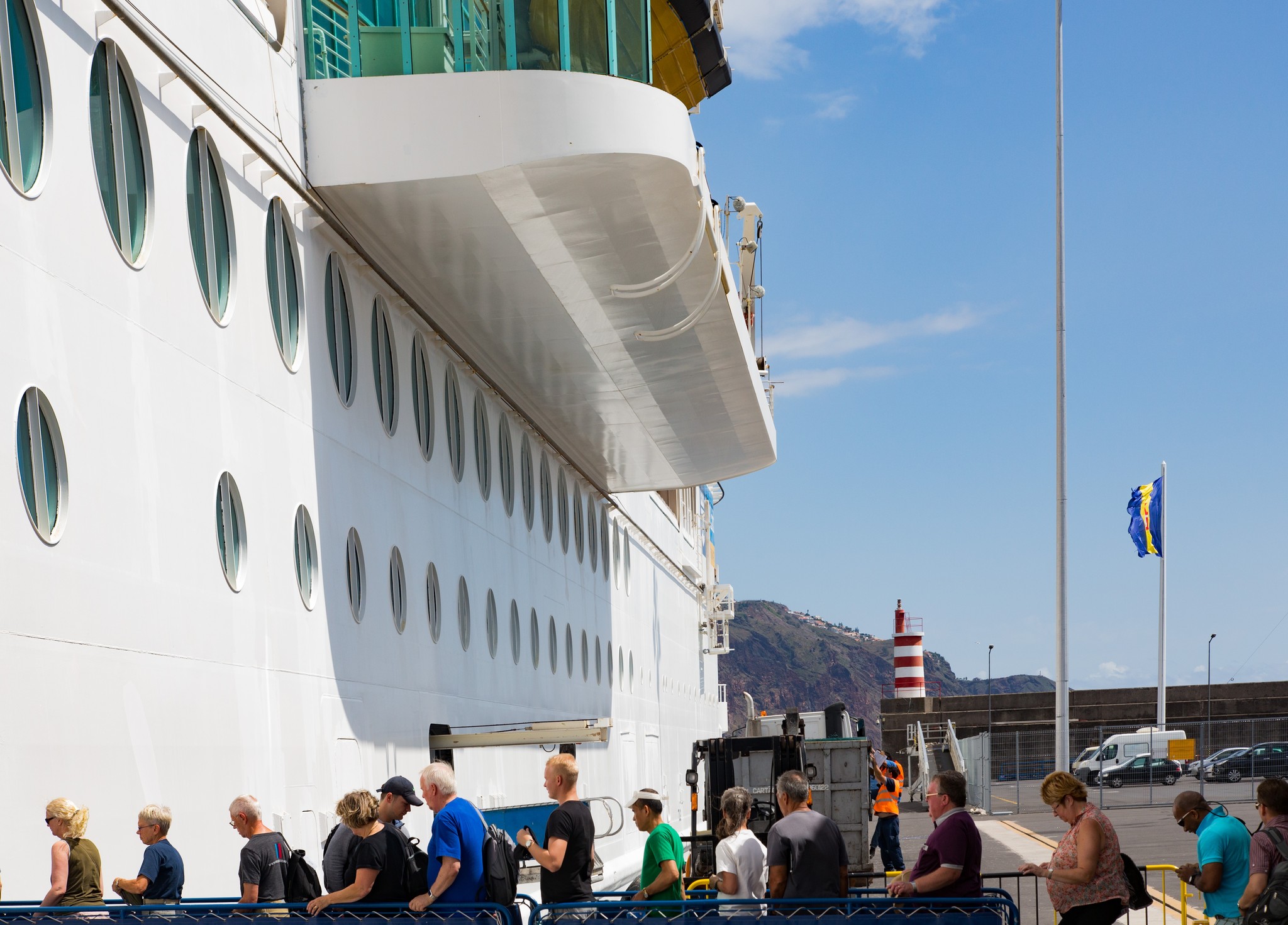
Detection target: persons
<box><xmin>409</xmin><ymin>759</ymin><xmax>490</xmax><ymax>918</ymax></box>
<box><xmin>1172</xmin><ymin>790</ymin><xmax>1251</xmax><ymax>925</ymax></box>
<box><xmin>624</xmin><ymin>788</ymin><xmax>688</xmax><ymax>919</ymax></box>
<box><xmin>1018</xmin><ymin>771</ymin><xmax>1132</xmax><ymax>925</ymax></box>
<box><xmin>31</xmin><ymin>798</ymin><xmax>112</xmax><ymax>920</ymax></box>
<box><xmin>321</xmin><ymin>774</ymin><xmax>424</xmax><ymax>894</ymax></box>
<box><xmin>885</xmin><ymin>767</ymin><xmax>982</xmax><ymax>913</ymax></box>
<box><xmin>870</xmin><ymin>740</ymin><xmax>903</xmax><ymax>858</ymax></box>
<box><xmin>766</xmin><ymin>770</ymin><xmax>850</xmax><ymax>917</ymax></box>
<box><xmin>1237</xmin><ymin>776</ymin><xmax>1288</xmax><ymax>925</ymax></box>
<box><xmin>112</xmin><ymin>803</ymin><xmax>186</xmax><ymax>919</ymax></box>
<box><xmin>707</xmin><ymin>786</ymin><xmax>768</xmax><ymax>916</ymax></box>
<box><xmin>229</xmin><ymin>794</ymin><xmax>290</xmax><ymax>917</ymax></box>
<box><xmin>516</xmin><ymin>752</ymin><xmax>598</xmax><ymax>919</ymax></box>
<box><xmin>307</xmin><ymin>790</ymin><xmax>419</xmax><ymax>916</ymax></box>
<box><xmin>869</xmin><ymin>753</ymin><xmax>905</xmax><ymax>872</ymax></box>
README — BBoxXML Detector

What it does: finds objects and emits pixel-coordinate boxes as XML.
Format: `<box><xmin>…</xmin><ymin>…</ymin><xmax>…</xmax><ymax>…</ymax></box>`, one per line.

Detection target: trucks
<box><xmin>678</xmin><ymin>690</ymin><xmax>876</xmax><ymax>900</ymax></box>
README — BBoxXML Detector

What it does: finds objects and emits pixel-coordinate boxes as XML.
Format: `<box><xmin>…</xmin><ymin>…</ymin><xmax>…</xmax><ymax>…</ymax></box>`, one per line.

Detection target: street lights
<box><xmin>1209</xmin><ymin>633</ymin><xmax>1216</xmax><ymax>758</ymax></box>
<box><xmin>988</xmin><ymin>645</ymin><xmax>993</xmax><ymax>733</ymax></box>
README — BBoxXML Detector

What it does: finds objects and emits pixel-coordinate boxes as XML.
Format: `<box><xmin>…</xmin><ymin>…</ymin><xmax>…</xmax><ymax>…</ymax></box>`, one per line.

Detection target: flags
<box><xmin>1127</xmin><ymin>477</ymin><xmax>1162</xmax><ymax>559</ymax></box>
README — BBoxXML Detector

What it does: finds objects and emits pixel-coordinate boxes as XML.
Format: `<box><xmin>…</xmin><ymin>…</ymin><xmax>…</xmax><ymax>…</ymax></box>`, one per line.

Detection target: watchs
<box><xmin>526</xmin><ymin>840</ymin><xmax>536</xmax><ymax>849</ymax></box>
<box><xmin>1237</xmin><ymin>900</ymin><xmax>1249</xmax><ymax>912</ymax></box>
<box><xmin>643</xmin><ymin>887</ymin><xmax>650</xmax><ymax>898</ymax></box>
<box><xmin>1048</xmin><ymin>868</ymin><xmax>1053</xmax><ymax>879</ymax></box>
<box><xmin>910</xmin><ymin>881</ymin><xmax>917</xmax><ymax>892</ymax></box>
<box><xmin>428</xmin><ymin>889</ymin><xmax>438</xmax><ymax>901</ymax></box>
<box><xmin>115</xmin><ymin>878</ymin><xmax>122</xmax><ymax>887</ymax></box>
<box><xmin>1190</xmin><ymin>873</ymin><xmax>1201</xmax><ymax>887</ymax></box>
<box><xmin>715</xmin><ymin>881</ymin><xmax>722</xmax><ymax>892</ymax></box>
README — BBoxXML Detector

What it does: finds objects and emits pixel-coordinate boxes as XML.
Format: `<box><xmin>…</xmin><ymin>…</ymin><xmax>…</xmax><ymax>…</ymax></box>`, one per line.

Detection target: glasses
<box><xmin>1255</xmin><ymin>802</ymin><xmax>1268</xmax><ymax>810</ymax></box>
<box><xmin>1052</xmin><ymin>795</ymin><xmax>1065</xmax><ymax>813</ymax></box>
<box><xmin>229</xmin><ymin>815</ymin><xmax>239</xmax><ymax>826</ymax></box>
<box><xmin>1177</xmin><ymin>807</ymin><xmax>1199</xmax><ymax>826</ymax></box>
<box><xmin>45</xmin><ymin>816</ymin><xmax>56</xmax><ymax>825</ymax></box>
<box><xmin>138</xmin><ymin>824</ymin><xmax>155</xmax><ymax>831</ymax></box>
<box><xmin>924</xmin><ymin>792</ymin><xmax>945</xmax><ymax>799</ymax></box>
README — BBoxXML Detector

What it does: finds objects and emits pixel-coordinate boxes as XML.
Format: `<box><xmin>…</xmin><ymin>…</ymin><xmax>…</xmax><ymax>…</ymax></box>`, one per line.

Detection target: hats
<box><xmin>624</xmin><ymin>791</ymin><xmax>669</xmax><ymax>808</ymax></box>
<box><xmin>513</xmin><ymin>825</ymin><xmax>539</xmax><ymax>861</ymax></box>
<box><xmin>376</xmin><ymin>776</ymin><xmax>424</xmax><ymax>806</ymax></box>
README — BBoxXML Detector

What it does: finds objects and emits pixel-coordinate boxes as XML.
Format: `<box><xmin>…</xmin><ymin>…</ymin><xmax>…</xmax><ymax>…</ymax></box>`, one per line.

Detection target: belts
<box><xmin>143</xmin><ymin>898</ymin><xmax>181</xmax><ymax>904</ymax></box>
<box><xmin>1214</xmin><ymin>914</ymin><xmax>1225</xmax><ymax>920</ymax></box>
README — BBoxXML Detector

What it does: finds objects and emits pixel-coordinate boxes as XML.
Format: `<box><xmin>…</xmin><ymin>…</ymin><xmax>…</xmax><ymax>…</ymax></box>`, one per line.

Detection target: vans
<box><xmin>1072</xmin><ymin>745</ymin><xmax>1113</xmax><ymax>778</ymax></box>
<box><xmin>1074</xmin><ymin>730</ymin><xmax>1190</xmax><ymax>787</ymax></box>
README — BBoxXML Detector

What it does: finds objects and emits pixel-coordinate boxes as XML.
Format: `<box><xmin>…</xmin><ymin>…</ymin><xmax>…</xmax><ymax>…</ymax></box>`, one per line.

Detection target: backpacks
<box><xmin>278</xmin><ymin>832</ymin><xmax>322</xmax><ymax>916</ymax></box>
<box><xmin>468</xmin><ymin>798</ymin><xmax>520</xmax><ymax>910</ymax></box>
<box><xmin>1242</xmin><ymin>826</ymin><xmax>1288</xmax><ymax>925</ymax></box>
<box><xmin>385</xmin><ymin>821</ymin><xmax>428</xmax><ymax>912</ymax></box>
<box><xmin>322</xmin><ymin>823</ymin><xmax>340</xmax><ymax>867</ymax></box>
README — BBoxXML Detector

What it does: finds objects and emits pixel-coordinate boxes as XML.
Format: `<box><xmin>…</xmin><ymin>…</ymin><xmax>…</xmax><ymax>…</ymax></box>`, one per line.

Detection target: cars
<box><xmin>1098</xmin><ymin>752</ymin><xmax>1182</xmax><ymax>789</ymax></box>
<box><xmin>1185</xmin><ymin>741</ymin><xmax>1288</xmax><ymax>783</ymax></box>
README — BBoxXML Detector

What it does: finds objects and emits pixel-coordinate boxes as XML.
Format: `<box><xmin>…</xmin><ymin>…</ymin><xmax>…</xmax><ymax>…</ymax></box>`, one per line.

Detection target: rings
<box><xmin>898</xmin><ymin>886</ymin><xmax>900</xmax><ymax>888</ymax></box>
<box><xmin>416</xmin><ymin>908</ymin><xmax>419</xmax><ymax>910</ymax></box>
<box><xmin>1029</xmin><ymin>865</ymin><xmax>1031</xmax><ymax>869</ymax></box>
<box><xmin>315</xmin><ymin>906</ymin><xmax>318</xmax><ymax>909</ymax></box>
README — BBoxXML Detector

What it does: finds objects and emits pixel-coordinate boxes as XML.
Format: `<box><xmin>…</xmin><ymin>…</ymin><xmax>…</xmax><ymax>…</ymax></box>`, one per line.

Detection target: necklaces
<box><xmin>365</xmin><ymin>821</ymin><xmax>380</xmax><ymax>838</ymax></box>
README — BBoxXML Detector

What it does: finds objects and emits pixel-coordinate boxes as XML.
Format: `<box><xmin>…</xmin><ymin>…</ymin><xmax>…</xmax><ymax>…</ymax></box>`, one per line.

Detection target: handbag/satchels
<box><xmin>120</xmin><ymin>889</ymin><xmax>142</xmax><ymax>905</ymax></box>
<box><xmin>1120</xmin><ymin>850</ymin><xmax>1153</xmax><ymax>911</ymax></box>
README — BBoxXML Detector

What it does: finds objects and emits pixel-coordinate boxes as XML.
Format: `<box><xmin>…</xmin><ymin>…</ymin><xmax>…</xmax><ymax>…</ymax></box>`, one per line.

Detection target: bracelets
<box><xmin>769</xmin><ymin>911</ymin><xmax>776</xmax><ymax>916</ymax></box>
